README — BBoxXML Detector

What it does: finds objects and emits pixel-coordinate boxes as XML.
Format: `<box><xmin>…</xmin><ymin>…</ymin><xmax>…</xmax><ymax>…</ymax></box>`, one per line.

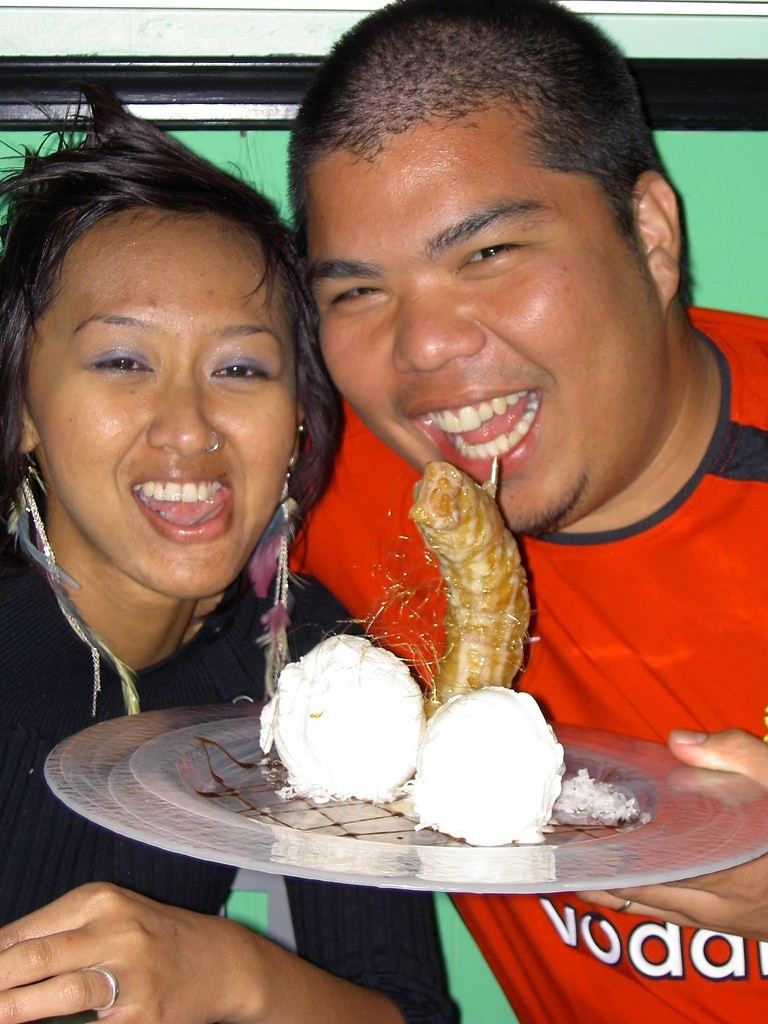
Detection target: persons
<box><xmin>289</xmin><ymin>1</ymin><xmax>768</xmax><ymax>1024</ymax></box>
<box><xmin>0</xmin><ymin>115</ymin><xmax>463</xmax><ymax>1024</ymax></box>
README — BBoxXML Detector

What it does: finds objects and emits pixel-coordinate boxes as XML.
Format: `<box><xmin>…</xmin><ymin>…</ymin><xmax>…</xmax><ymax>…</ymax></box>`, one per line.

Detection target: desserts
<box><xmin>409</xmin><ymin>683</ymin><xmax>564</xmax><ymax>846</ymax></box>
<box><xmin>408</xmin><ymin>460</ymin><xmax>529</xmax><ymax>715</ymax></box>
<box><xmin>258</xmin><ymin>635</ymin><xmax>424</xmax><ymax>800</ymax></box>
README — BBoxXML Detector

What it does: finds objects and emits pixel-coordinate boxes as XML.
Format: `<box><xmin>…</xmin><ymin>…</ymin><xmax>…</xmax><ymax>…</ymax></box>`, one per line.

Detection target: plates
<box><xmin>44</xmin><ymin>700</ymin><xmax>767</xmax><ymax>893</ymax></box>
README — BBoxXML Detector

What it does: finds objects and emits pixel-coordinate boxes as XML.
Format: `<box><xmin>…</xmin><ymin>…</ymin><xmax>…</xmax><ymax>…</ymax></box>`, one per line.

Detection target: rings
<box><xmin>86</xmin><ymin>964</ymin><xmax>120</xmax><ymax>1012</ymax></box>
<box><xmin>611</xmin><ymin>900</ymin><xmax>632</xmax><ymax>913</ymax></box>
